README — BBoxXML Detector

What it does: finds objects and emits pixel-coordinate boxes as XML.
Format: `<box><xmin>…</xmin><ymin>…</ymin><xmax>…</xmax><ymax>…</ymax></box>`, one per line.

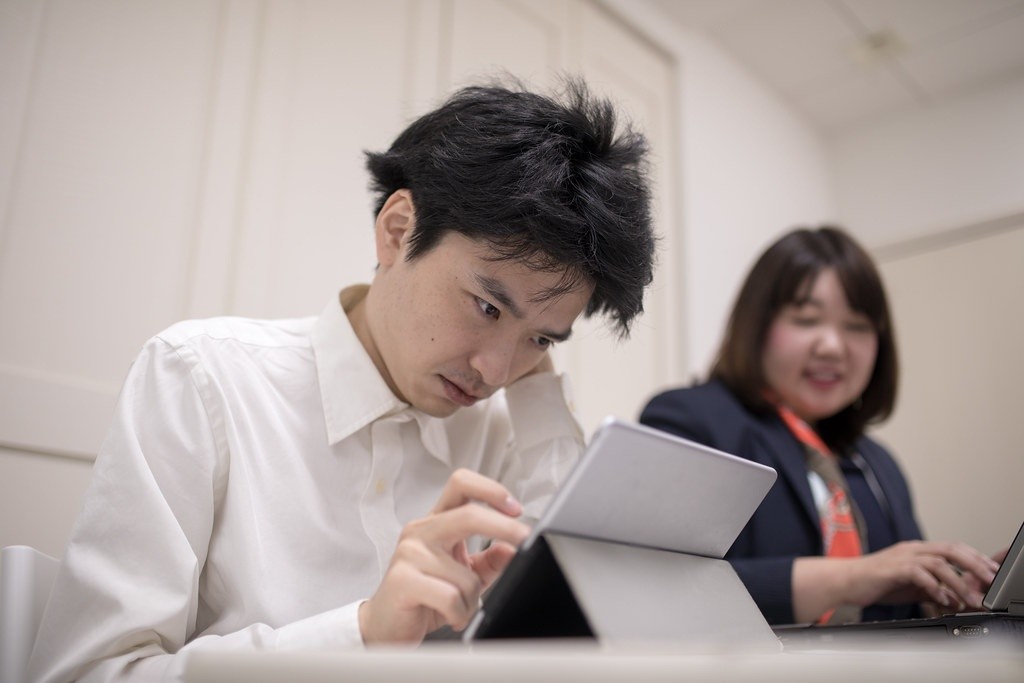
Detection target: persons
<box><xmin>26</xmin><ymin>85</ymin><xmax>656</xmax><ymax>683</ymax></box>
<box><xmin>641</xmin><ymin>222</ymin><xmax>1007</xmax><ymax>628</ymax></box>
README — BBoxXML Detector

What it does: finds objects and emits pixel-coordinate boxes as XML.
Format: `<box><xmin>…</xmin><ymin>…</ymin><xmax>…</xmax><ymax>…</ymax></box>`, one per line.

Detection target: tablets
<box><xmin>460</xmin><ymin>418</ymin><xmax>775</xmax><ymax>643</ymax></box>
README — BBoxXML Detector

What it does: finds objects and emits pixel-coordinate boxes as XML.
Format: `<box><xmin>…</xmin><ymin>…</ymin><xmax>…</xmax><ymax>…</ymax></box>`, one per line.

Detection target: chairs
<box><xmin>0</xmin><ymin>545</ymin><xmax>59</xmax><ymax>682</ymax></box>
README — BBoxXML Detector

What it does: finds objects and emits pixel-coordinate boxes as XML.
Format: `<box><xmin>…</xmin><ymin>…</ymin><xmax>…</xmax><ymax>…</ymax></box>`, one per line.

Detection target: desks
<box><xmin>184</xmin><ymin>627</ymin><xmax>1024</xmax><ymax>681</ymax></box>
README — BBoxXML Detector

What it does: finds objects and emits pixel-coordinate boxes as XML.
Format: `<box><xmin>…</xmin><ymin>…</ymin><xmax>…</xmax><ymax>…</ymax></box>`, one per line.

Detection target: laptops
<box><xmin>775</xmin><ymin>522</ymin><xmax>1024</xmax><ymax>632</ymax></box>
